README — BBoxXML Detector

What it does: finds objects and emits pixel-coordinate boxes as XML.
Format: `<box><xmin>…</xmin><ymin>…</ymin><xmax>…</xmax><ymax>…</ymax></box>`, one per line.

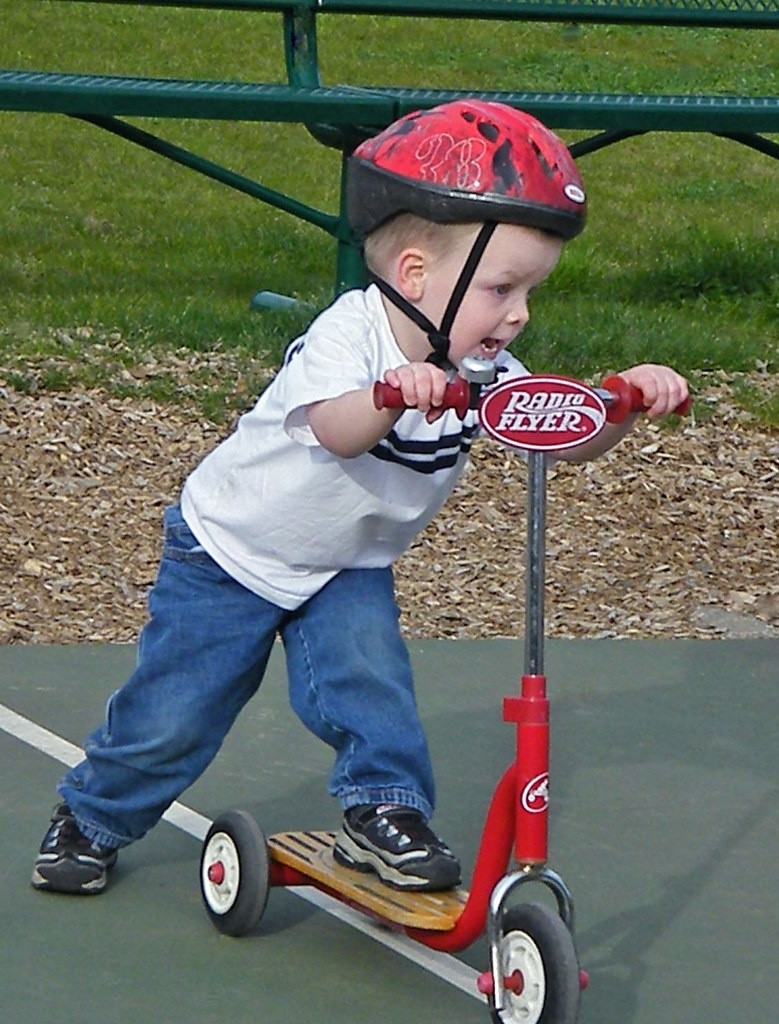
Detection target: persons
<box><xmin>30</xmin><ymin>98</ymin><xmax>690</xmax><ymax>896</ymax></box>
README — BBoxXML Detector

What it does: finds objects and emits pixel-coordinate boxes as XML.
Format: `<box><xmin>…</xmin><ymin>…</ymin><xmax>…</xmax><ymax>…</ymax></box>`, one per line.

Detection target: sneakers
<box><xmin>332</xmin><ymin>802</ymin><xmax>462</xmax><ymax>893</ymax></box>
<box><xmin>32</xmin><ymin>802</ymin><xmax>118</xmax><ymax>896</ymax></box>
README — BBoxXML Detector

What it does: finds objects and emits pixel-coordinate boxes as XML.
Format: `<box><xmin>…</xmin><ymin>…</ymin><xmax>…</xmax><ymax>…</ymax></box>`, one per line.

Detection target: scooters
<box><xmin>195</xmin><ymin>353</ymin><xmax>697</xmax><ymax>1024</ymax></box>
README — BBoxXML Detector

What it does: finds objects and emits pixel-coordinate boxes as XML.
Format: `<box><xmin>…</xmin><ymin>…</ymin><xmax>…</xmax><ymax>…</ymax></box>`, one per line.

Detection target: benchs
<box><xmin>0</xmin><ymin>0</ymin><xmax>779</xmax><ymax>316</ymax></box>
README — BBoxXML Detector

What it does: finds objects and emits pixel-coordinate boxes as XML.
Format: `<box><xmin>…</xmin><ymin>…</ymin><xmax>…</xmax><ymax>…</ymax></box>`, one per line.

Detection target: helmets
<box><xmin>343</xmin><ymin>99</ymin><xmax>588</xmax><ymax>243</ymax></box>
